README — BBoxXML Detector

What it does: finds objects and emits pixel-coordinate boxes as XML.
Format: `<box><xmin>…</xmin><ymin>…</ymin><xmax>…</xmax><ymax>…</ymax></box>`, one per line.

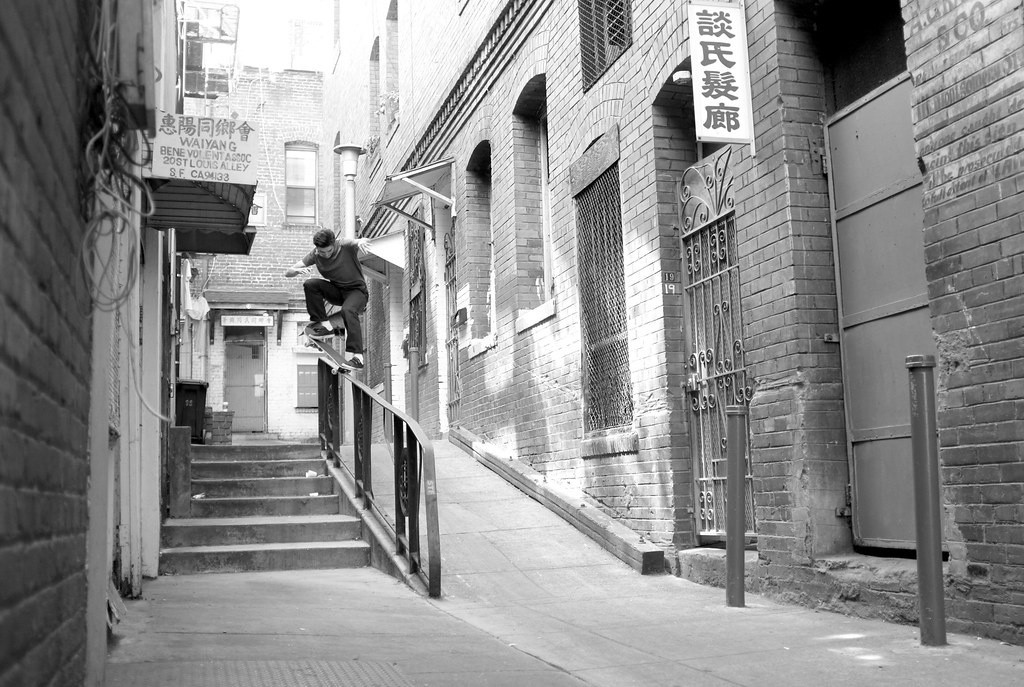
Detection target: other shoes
<box><xmin>341</xmin><ymin>357</ymin><xmax>366</xmax><ymax>372</ymax></box>
<box><xmin>308</xmin><ymin>326</ymin><xmax>339</xmax><ymax>340</ymax></box>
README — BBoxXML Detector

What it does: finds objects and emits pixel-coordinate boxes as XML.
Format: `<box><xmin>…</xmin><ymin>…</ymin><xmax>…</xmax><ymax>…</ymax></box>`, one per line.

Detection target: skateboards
<box><xmin>304</xmin><ymin>336</ymin><xmax>351</xmax><ymax>375</ymax></box>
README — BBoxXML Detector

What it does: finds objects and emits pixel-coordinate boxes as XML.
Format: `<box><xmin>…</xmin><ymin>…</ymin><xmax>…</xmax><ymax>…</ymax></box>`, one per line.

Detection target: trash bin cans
<box><xmin>175</xmin><ymin>377</ymin><xmax>209</xmax><ymax>444</ymax></box>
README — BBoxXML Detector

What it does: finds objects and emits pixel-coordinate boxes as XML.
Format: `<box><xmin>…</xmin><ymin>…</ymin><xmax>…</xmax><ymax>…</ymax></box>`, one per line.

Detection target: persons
<box><xmin>285</xmin><ymin>227</ymin><xmax>373</xmax><ymax>371</ymax></box>
